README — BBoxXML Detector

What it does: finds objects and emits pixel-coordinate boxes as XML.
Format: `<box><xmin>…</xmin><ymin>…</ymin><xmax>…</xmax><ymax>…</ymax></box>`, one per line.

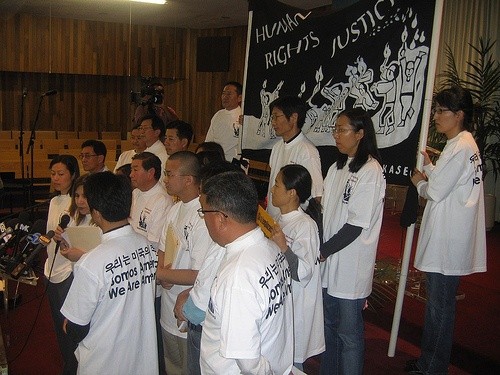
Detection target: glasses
<box><xmin>432</xmin><ymin>109</ymin><xmax>451</xmax><ymax>114</ymax></box>
<box><xmin>164</xmin><ymin>172</ymin><xmax>197</xmax><ymax>178</ymax></box>
<box><xmin>197</xmin><ymin>208</ymin><xmax>228</xmax><ymax>218</ymax></box>
<box><xmin>331</xmin><ymin>129</ymin><xmax>357</xmax><ymax>133</ymax></box>
<box><xmin>138</xmin><ymin>127</ymin><xmax>154</xmax><ymax>131</ymax></box>
<box><xmin>270</xmin><ymin>114</ymin><xmax>285</xmax><ymax>120</ymax></box>
<box><xmin>79</xmin><ymin>154</ymin><xmax>101</xmax><ymax>158</ymax></box>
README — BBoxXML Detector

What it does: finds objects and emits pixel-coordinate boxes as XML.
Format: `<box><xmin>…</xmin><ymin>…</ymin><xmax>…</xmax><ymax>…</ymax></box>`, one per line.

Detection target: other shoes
<box><xmin>404</xmin><ymin>360</ymin><xmax>422</xmax><ymax>375</ymax></box>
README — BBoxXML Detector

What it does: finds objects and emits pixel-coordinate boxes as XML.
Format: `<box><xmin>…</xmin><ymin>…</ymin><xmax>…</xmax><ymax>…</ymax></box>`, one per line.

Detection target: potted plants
<box><xmin>427</xmin><ymin>34</ymin><xmax>500</xmax><ymax>232</ymax></box>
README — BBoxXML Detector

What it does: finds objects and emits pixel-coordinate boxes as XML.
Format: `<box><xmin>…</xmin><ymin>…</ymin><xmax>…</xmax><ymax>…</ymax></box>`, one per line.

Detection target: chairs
<box><xmin>0</xmin><ymin>130</ymin><xmax>202</xmax><ymax>203</ymax></box>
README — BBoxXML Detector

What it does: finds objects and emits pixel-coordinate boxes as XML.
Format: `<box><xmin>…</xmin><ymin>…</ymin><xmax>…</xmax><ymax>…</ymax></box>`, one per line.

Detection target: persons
<box><xmin>320</xmin><ymin>107</ymin><xmax>387</xmax><ymax>375</ymax></box>
<box><xmin>266</xmin><ymin>94</ymin><xmax>323</xmax><ymax>219</ymax></box>
<box><xmin>406</xmin><ymin>89</ymin><xmax>487</xmax><ymax>375</ymax></box>
<box><xmin>44</xmin><ymin>82</ymin><xmax>326</xmax><ymax>375</ymax></box>
<box><xmin>204</xmin><ymin>82</ymin><xmax>249</xmax><ymax>175</ymax></box>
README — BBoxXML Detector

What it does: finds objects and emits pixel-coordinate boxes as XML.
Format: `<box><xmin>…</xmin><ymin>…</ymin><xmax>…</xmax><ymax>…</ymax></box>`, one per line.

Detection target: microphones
<box><xmin>42</xmin><ymin>90</ymin><xmax>58</xmax><ymax>96</ymax></box>
<box><xmin>0</xmin><ymin>217</ymin><xmax>55</xmax><ymax>280</ymax></box>
<box><xmin>55</xmin><ymin>214</ymin><xmax>70</xmax><ymax>254</ymax></box>
<box><xmin>22</xmin><ymin>88</ymin><xmax>28</xmax><ymax>98</ymax></box>
